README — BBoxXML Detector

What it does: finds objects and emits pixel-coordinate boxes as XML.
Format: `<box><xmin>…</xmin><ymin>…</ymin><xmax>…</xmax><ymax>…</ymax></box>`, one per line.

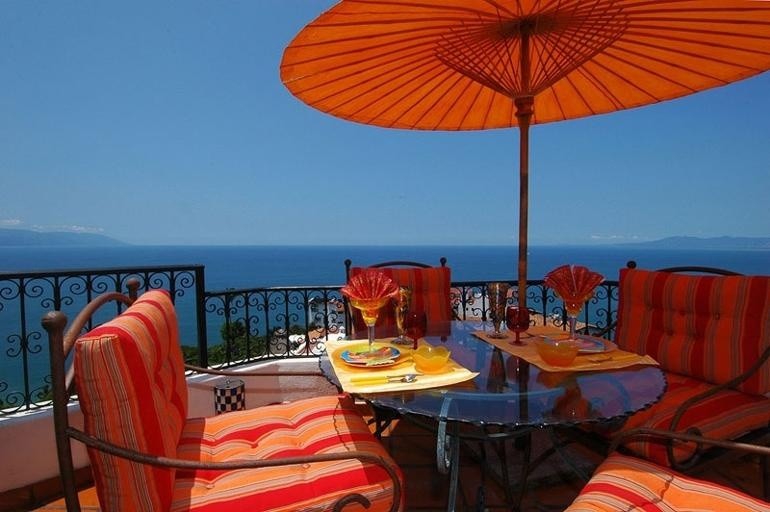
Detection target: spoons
<box><xmin>352</xmin><ymin>376</ymin><xmax>417</xmax><ymax>387</ymax></box>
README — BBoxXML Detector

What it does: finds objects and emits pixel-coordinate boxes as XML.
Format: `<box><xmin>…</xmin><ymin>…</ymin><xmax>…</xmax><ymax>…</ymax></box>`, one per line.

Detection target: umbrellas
<box><xmin>278</xmin><ymin>0</ymin><xmax>770</xmax><ymax>449</ymax></box>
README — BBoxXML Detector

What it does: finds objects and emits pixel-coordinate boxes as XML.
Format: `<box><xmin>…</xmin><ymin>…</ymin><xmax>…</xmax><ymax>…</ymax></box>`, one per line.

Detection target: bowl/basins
<box><xmin>535</xmin><ymin>341</ymin><xmax>579</xmax><ymax>368</ymax></box>
<box><xmin>409</xmin><ymin>345</ymin><xmax>452</xmax><ymax>373</ymax></box>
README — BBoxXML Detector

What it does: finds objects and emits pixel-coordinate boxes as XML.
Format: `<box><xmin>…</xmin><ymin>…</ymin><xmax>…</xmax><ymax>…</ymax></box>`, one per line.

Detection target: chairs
<box><xmin>563</xmin><ymin>429</ymin><xmax>769</xmax><ymax>511</ymax></box>
<box><xmin>39</xmin><ymin>277</ymin><xmax>404</xmax><ymax>512</ymax></box>
<box><xmin>554</xmin><ymin>261</ymin><xmax>769</xmax><ymax>474</ymax></box>
<box><xmin>344</xmin><ymin>255</ymin><xmax>486</xmax><ymax>465</ymax></box>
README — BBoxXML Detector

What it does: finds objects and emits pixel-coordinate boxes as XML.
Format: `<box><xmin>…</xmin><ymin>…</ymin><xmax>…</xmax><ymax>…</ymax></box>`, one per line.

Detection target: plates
<box><xmin>544</xmin><ymin>333</ymin><xmax>618</xmax><ymax>355</ymax></box>
<box><xmin>341</xmin><ymin>346</ymin><xmax>401</xmax><ymax>363</ymax></box>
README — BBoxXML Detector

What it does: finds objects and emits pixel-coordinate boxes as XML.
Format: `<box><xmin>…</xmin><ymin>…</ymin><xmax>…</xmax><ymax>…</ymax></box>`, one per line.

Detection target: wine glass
<box><xmin>405</xmin><ymin>313</ymin><xmax>427</xmax><ymax>350</ymax></box>
<box><xmin>390</xmin><ymin>283</ymin><xmax>413</xmax><ymax>344</ymax></box>
<box><xmin>486</xmin><ymin>282</ymin><xmax>509</xmax><ymax>340</ymax></box>
<box><xmin>563</xmin><ymin>298</ymin><xmax>584</xmax><ymax>341</ymax></box>
<box><xmin>505</xmin><ymin>305</ymin><xmax>532</xmax><ymax>348</ymax></box>
<box><xmin>349</xmin><ymin>293</ymin><xmax>392</xmax><ymax>355</ymax></box>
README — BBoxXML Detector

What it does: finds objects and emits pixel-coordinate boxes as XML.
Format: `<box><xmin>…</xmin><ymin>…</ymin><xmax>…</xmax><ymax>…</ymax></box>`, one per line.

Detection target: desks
<box><xmin>319</xmin><ymin>318</ymin><xmax>667</xmax><ymax>508</ymax></box>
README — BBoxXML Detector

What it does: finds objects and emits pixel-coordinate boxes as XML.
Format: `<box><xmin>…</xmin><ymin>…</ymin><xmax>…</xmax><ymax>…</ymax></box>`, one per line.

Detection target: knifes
<box><xmin>350</xmin><ymin>373</ymin><xmax>423</xmax><ymax>381</ymax></box>
